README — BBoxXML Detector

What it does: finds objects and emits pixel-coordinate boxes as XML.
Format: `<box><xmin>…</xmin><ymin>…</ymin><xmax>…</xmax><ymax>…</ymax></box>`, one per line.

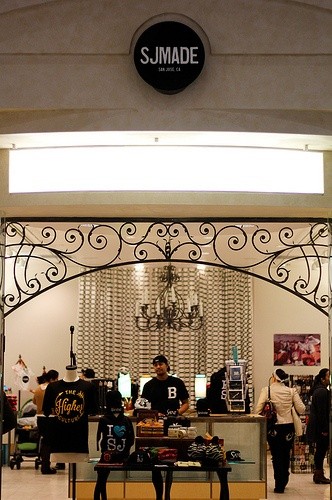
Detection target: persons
<box><xmin>141</xmin><ymin>354</ymin><xmax>190</xmax><ymax>415</ymax></box>
<box><xmin>33</xmin><ymin>370</ymin><xmax>60</xmax><ymax>474</ymax></box>
<box><xmin>305</xmin><ymin>369</ymin><xmax>332</xmax><ymax>484</ymax></box>
<box><xmin>80</xmin><ymin>368</ymin><xmax>95</xmax><ymax>380</ymax></box>
<box><xmin>255</xmin><ymin>369</ymin><xmax>306</xmax><ymax>493</ymax></box>
<box><xmin>274</xmin><ymin>336</ymin><xmax>321</xmax><ymax>366</ymax></box>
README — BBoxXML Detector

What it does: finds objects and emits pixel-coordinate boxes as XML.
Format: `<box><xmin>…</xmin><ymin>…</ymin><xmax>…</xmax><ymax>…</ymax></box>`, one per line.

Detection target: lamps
<box><xmin>133</xmin><ymin>262</ymin><xmax>204</xmax><ymax>333</ymax></box>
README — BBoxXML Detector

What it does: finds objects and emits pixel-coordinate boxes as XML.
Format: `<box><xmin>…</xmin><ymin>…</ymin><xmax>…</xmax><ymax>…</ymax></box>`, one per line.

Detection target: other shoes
<box><xmin>274</xmin><ymin>487</ymin><xmax>284</xmax><ymax>493</ymax></box>
<box><xmin>52</xmin><ymin>465</ymin><xmax>65</xmax><ymax>470</ymax></box>
<box><xmin>41</xmin><ymin>468</ymin><xmax>55</xmax><ymax>473</ymax></box>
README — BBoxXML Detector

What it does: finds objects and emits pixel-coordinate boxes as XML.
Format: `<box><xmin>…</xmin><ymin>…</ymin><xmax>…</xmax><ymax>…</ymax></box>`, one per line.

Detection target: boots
<box><xmin>313</xmin><ymin>469</ymin><xmax>331</xmax><ymax>484</ymax></box>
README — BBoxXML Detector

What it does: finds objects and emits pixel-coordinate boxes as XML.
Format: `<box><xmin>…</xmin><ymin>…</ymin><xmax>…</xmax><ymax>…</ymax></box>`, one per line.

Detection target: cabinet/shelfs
<box><xmin>94</xmin><ymin>436</ymin><xmax>232</xmax><ymax>500</ymax></box>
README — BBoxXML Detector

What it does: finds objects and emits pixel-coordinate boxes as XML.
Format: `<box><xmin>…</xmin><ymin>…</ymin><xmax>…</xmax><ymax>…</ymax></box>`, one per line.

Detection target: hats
<box><xmin>188</xmin><ymin>436</ymin><xmax>206</xmax><ymax>460</ymax></box>
<box><xmin>226</xmin><ymin>450</ymin><xmax>245</xmax><ymax>461</ymax></box>
<box><xmin>205</xmin><ymin>435</ymin><xmax>226</xmax><ymax>462</ymax></box>
<box><xmin>81</xmin><ymin>368</ymin><xmax>95</xmax><ymax>379</ymax></box>
<box><xmin>153</xmin><ymin>356</ymin><xmax>170</xmax><ymax>373</ymax></box>
<box><xmin>127</xmin><ymin>450</ymin><xmax>152</xmax><ymax>467</ymax></box>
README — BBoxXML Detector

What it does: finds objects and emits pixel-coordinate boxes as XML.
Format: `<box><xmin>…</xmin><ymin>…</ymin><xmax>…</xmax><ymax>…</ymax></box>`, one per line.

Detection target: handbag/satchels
<box><xmin>262</xmin><ymin>387</ymin><xmax>277</xmax><ymax>428</ymax></box>
<box><xmin>291</xmin><ymin>389</ymin><xmax>303</xmax><ymax>436</ymax></box>
